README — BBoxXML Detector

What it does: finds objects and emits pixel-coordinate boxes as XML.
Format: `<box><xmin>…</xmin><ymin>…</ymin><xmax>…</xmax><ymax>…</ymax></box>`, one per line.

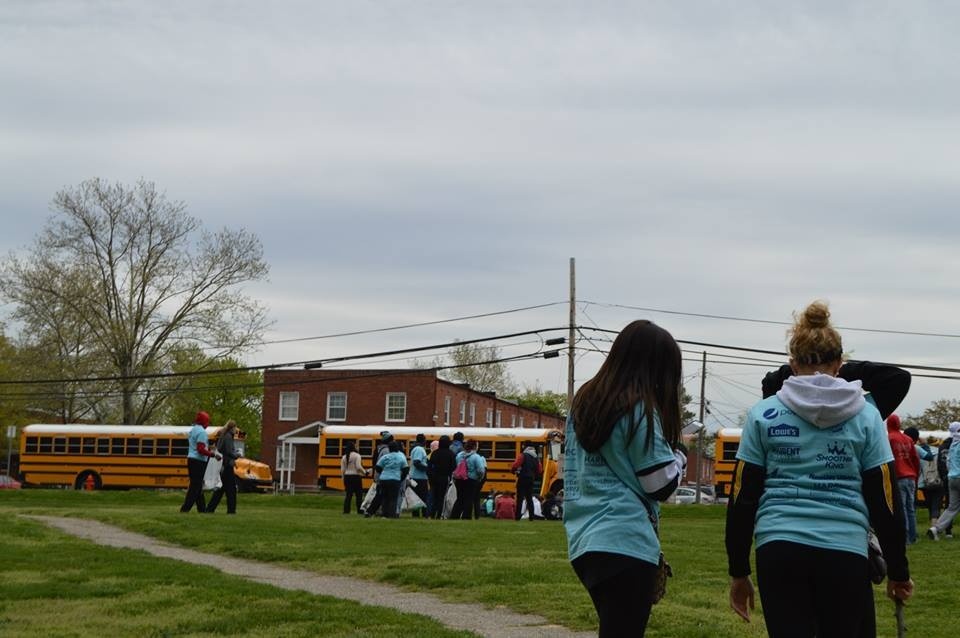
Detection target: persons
<box><xmin>510</xmin><ymin>440</ymin><xmax>539</xmax><ymax>522</ymax></box>
<box><xmin>341</xmin><ymin>432</ymin><xmax>488</xmax><ymax>520</ymax></box>
<box><xmin>480</xmin><ymin>488</ymin><xmax>498</xmax><ymax>518</ymax></box>
<box><xmin>492</xmin><ymin>486</ymin><xmax>563</xmax><ymax>520</ymax></box>
<box><xmin>180</xmin><ymin>411</ymin><xmax>222</xmax><ymax>513</ymax></box>
<box><xmin>725</xmin><ymin>300</ymin><xmax>914</xmax><ymax>638</ymax></box>
<box><xmin>207</xmin><ymin>419</ymin><xmax>241</xmax><ymax>514</ymax></box>
<box><xmin>760</xmin><ymin>355</ymin><xmax>960</xmax><ymax>543</ymax></box>
<box><xmin>563</xmin><ymin>320</ymin><xmax>699</xmax><ymax>638</ymax></box>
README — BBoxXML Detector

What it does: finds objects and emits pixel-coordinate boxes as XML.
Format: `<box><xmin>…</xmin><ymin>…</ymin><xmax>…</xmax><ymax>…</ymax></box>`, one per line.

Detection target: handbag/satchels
<box><xmin>202</xmin><ymin>450</ymin><xmax>222</xmax><ymax>490</ymax></box>
<box><xmin>865</xmin><ymin>530</ymin><xmax>887</xmax><ymax>585</ymax></box>
<box><xmin>652</xmin><ymin>552</ymin><xmax>672</xmax><ymax>604</ymax></box>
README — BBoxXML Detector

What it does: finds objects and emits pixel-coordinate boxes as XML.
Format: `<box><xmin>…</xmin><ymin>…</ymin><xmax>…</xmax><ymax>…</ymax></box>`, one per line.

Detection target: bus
<box><xmin>20</xmin><ymin>420</ymin><xmax>275</xmax><ymax>495</ymax></box>
<box><xmin>712</xmin><ymin>425</ymin><xmax>956</xmax><ymax>504</ymax></box>
<box><xmin>317</xmin><ymin>422</ymin><xmax>561</xmax><ymax>505</ymax></box>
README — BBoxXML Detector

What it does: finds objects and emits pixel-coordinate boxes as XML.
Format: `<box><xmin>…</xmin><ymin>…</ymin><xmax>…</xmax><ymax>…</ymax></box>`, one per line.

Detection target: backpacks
<box><xmin>452</xmin><ymin>459</ymin><xmax>468</xmax><ymax>480</ymax></box>
<box><xmin>923</xmin><ymin>454</ymin><xmax>941</xmax><ymax>486</ymax></box>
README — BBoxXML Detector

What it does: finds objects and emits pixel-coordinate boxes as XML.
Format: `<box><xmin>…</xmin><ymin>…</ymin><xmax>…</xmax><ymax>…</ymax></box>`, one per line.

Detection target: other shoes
<box><xmin>945</xmin><ymin>534</ymin><xmax>952</xmax><ymax>539</ymax></box>
<box><xmin>926</xmin><ymin>527</ymin><xmax>939</xmax><ymax>541</ymax></box>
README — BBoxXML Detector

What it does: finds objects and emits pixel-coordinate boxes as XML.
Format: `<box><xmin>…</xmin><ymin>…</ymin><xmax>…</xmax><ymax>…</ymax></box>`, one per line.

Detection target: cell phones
<box><xmin>681</xmin><ymin>421</ymin><xmax>704</xmax><ymax>435</ymax></box>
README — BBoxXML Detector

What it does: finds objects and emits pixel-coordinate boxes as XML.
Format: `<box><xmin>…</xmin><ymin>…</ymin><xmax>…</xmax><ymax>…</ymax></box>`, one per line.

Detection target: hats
<box><xmin>379</xmin><ymin>431</ymin><xmax>392</xmax><ymax>439</ymax></box>
<box><xmin>194</xmin><ymin>411</ymin><xmax>210</xmax><ymax>426</ymax></box>
